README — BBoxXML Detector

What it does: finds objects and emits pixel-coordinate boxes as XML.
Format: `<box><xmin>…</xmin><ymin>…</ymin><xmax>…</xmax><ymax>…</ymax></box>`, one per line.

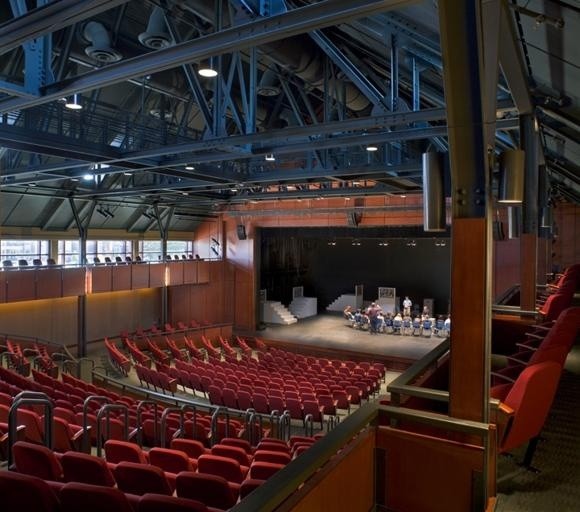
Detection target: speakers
<box><xmin>346</xmin><ymin>211</ymin><xmax>358</xmax><ymax>229</ymax></box>
<box><xmin>236</xmin><ymin>224</ymin><xmax>246</xmax><ymax>240</ymax></box>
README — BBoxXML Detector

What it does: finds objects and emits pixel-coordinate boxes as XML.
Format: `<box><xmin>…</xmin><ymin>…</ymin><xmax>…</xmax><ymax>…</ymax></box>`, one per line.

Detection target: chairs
<box><xmin>347</xmin><ymin>311</ymin><xmax>451</xmax><ymax>339</ymax></box>
<box><xmin>3</xmin><ymin>252</ymin><xmax>201</xmax><ymax>273</ymax></box>
<box><xmin>532</xmin><ymin>264</ymin><xmax>580</xmax><ymax>318</ymax></box>
<box><xmin>0</xmin><ymin>319</ymin><xmax>386</xmax><ymax>512</ymax></box>
<box><xmin>416</xmin><ymin>304</ymin><xmax>580</xmax><ymax>500</ymax></box>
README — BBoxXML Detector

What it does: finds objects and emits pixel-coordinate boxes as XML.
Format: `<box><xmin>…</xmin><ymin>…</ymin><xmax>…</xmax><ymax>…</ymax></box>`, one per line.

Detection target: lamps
<box><xmin>81</xmin><ymin>172</ymin><xmax>96</xmax><ymax>182</ymax></box>
<box><xmin>553</xmin><ymin>227</ymin><xmax>559</xmax><ymax>238</ymax></box>
<box><xmin>540</xmin><ymin>206</ymin><xmax>552</xmax><ymax>228</ymax></box>
<box><xmin>263</xmin><ymin>153</ymin><xmax>276</xmax><ymax>164</ymax></box>
<box><xmin>421</xmin><ymin>153</ymin><xmax>446</xmax><ymax>232</ymax></box>
<box><xmin>363</xmin><ymin>143</ymin><xmax>379</xmax><ymax>153</ymax></box>
<box><xmin>498</xmin><ymin>151</ymin><xmax>526</xmax><ymax>205</ymax></box>
<box><xmin>198</xmin><ymin>57</ymin><xmax>219</xmax><ymax>77</ymax></box>
<box><xmin>65</xmin><ymin>94</ymin><xmax>83</xmax><ymax>109</ymax></box>
<box><xmin>508</xmin><ymin>206</ymin><xmax>521</xmax><ymax>239</ymax></box>
<box><xmin>124</xmin><ymin>170</ymin><xmax>135</xmax><ymax>179</ymax></box>
<box><xmin>95</xmin><ymin>203</ymin><xmax>159</xmax><ymax>220</ymax></box>
<box><xmin>185</xmin><ymin>163</ymin><xmax>194</xmax><ymax>170</ymax></box>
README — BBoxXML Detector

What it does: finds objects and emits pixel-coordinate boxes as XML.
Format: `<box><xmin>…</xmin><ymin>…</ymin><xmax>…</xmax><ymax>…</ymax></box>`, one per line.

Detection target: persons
<box><xmin>342</xmin><ymin>295</ymin><xmax>451</xmax><ymax>339</ymax></box>
